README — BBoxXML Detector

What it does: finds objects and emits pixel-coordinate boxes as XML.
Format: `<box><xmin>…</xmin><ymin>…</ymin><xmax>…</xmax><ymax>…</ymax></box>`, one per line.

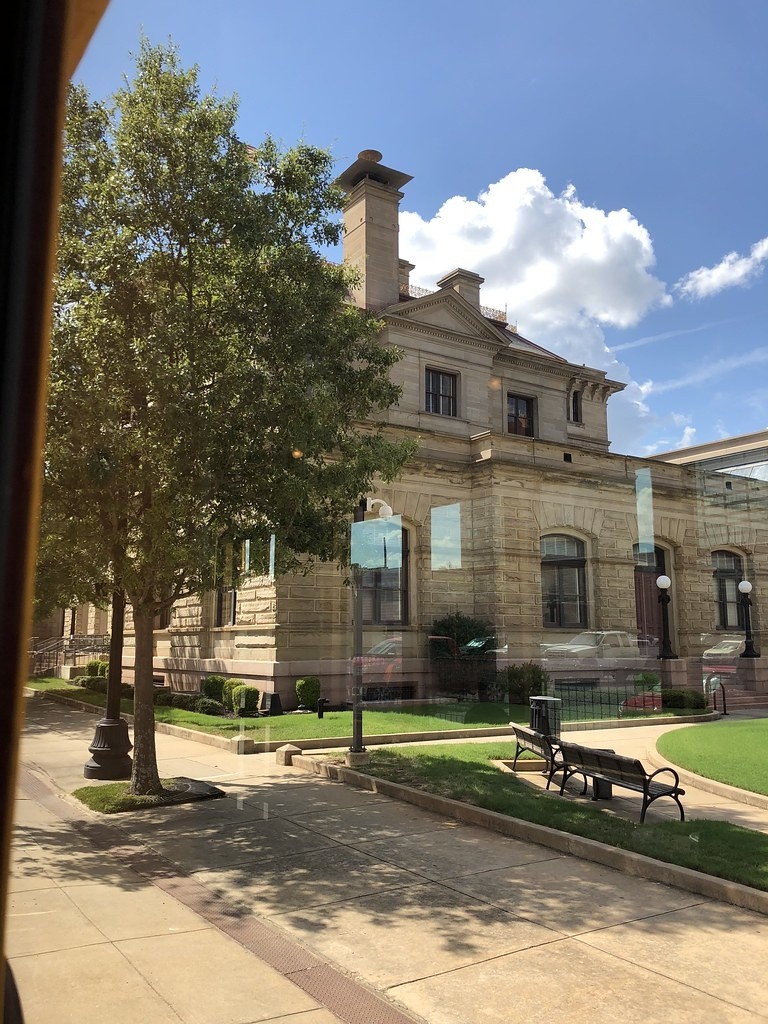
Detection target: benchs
<box><xmin>556</xmin><ymin>740</ymin><xmax>685</xmax><ymax>825</ymax></box>
<box><xmin>509</xmin><ymin>722</ymin><xmax>588</xmax><ymax>795</ymax></box>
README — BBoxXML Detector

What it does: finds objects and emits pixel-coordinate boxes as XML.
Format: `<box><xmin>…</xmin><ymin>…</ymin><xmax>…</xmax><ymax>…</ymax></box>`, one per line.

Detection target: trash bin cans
<box><xmin>528</xmin><ymin>695</ymin><xmax>562</xmax><ymax>743</ymax></box>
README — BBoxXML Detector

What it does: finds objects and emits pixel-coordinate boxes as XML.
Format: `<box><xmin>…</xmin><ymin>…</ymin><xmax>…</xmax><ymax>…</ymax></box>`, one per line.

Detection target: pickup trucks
<box><xmin>545</xmin><ymin>631</ymin><xmax>662</xmax><ymax>658</ymax></box>
<box><xmin>647</xmin><ymin>635</ymin><xmax>658</xmax><ymax>641</ymax></box>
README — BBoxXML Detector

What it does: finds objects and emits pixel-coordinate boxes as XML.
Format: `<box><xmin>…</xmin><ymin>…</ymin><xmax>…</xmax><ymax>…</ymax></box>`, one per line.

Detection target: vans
<box><xmin>633</xmin><ymin>639</ymin><xmax>652</xmax><ymax>654</ymax></box>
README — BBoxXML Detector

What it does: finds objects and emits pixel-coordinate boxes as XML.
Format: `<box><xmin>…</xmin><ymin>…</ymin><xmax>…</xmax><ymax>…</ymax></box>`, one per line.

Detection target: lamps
<box><xmin>656</xmin><ymin>575</ymin><xmax>672</xmax><ymax>589</ymax></box>
<box><xmin>738</xmin><ymin>581</ymin><xmax>753</xmax><ymax>593</ymax></box>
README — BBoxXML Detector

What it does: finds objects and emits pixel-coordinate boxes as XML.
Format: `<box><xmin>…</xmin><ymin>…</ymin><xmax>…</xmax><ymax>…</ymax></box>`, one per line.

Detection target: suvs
<box><xmin>701</xmin><ymin>632</ymin><xmax>712</xmax><ymax>641</ymax></box>
<box><xmin>702</xmin><ymin>638</ymin><xmax>748</xmax><ymax>661</ymax></box>
<box><xmin>459</xmin><ymin>636</ymin><xmax>496</xmax><ymax>656</ymax></box>
<box><xmin>347</xmin><ymin>636</ymin><xmax>461</xmax><ymax>683</ymax></box>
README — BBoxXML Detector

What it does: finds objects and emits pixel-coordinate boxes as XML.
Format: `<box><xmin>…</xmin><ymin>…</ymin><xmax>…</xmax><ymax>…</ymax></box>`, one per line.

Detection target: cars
<box><xmin>487</xmin><ymin>644</ymin><xmax>508</xmax><ymax>657</ymax></box>
<box><xmin>619</xmin><ymin>666</ymin><xmax>736</xmax><ymax>716</ymax></box>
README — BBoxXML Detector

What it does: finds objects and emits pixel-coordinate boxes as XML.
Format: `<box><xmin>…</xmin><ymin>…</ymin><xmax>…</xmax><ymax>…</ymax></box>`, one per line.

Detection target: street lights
<box><xmin>656</xmin><ymin>575</ymin><xmax>680</xmax><ymax>664</ymax></box>
<box><xmin>739</xmin><ymin>580</ymin><xmax>760</xmax><ymax>657</ymax></box>
